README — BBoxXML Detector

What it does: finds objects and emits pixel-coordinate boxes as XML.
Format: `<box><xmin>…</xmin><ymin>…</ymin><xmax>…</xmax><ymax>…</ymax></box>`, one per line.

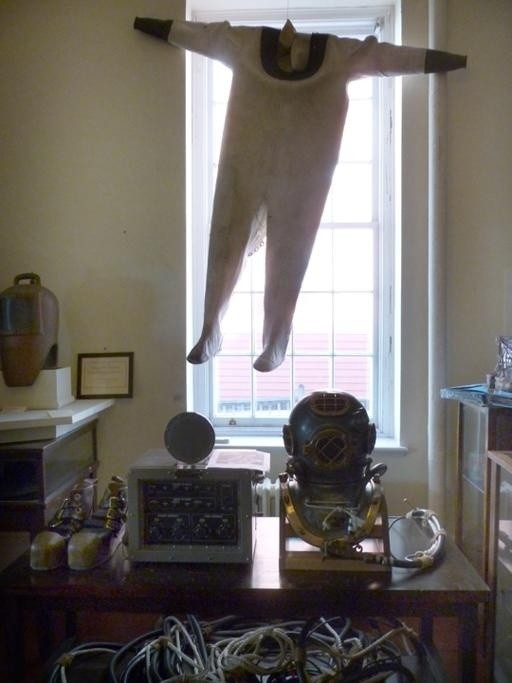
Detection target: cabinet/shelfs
<box><xmin>486</xmin><ymin>450</ymin><xmax>512</xmax><ymax>683</ymax></box>
<box><xmin>451</xmin><ymin>398</ymin><xmax>512</xmax><ymax>660</ymax></box>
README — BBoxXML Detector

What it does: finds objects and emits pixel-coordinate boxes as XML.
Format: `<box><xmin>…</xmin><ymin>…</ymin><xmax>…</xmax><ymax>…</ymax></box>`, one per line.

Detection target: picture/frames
<box><xmin>77</xmin><ymin>352</ymin><xmax>134</xmax><ymax>400</ymax></box>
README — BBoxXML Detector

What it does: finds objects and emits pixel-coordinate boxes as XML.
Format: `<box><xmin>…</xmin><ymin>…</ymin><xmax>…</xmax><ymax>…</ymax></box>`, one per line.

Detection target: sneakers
<box><xmin>29</xmin><ymin>478</ymin><xmax>99</xmax><ymax>571</ymax></box>
<box><xmin>69</xmin><ymin>475</ymin><xmax>130</xmax><ymax>573</ymax></box>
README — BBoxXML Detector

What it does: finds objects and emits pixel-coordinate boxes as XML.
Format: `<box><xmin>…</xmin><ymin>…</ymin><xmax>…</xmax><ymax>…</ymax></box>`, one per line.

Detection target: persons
<box><xmin>131</xmin><ymin>15</ymin><xmax>469</xmax><ymax>373</ymax></box>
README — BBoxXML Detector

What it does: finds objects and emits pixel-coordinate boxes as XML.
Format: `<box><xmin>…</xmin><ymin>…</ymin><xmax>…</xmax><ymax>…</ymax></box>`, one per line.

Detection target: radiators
<box><xmin>252</xmin><ymin>474</ymin><xmax>281</xmax><ymax>516</ymax></box>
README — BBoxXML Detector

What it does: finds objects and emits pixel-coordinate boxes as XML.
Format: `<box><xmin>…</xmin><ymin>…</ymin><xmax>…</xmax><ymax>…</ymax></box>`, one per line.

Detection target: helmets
<box><xmin>282</xmin><ymin>390</ymin><xmax>377</xmax><ymax>484</ymax></box>
<box><xmin>0</xmin><ymin>272</ymin><xmax>59</xmax><ymax>388</ymax></box>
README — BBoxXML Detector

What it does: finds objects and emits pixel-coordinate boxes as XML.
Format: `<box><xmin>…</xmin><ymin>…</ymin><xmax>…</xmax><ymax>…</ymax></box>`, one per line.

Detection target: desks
<box><xmin>12</xmin><ymin>516</ymin><xmax>490</xmax><ymax>683</ymax></box>
